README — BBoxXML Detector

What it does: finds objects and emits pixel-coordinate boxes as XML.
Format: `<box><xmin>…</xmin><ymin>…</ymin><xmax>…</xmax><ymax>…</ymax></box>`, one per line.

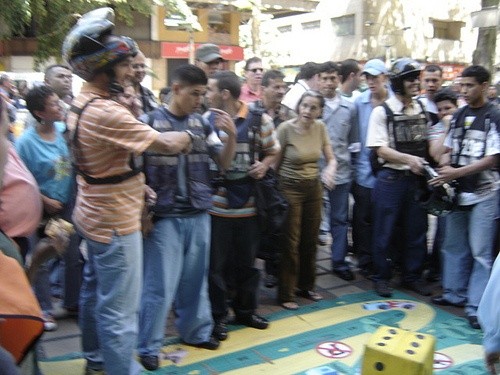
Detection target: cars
<box><xmin>448</xmin><ymin>74</ymin><xmax>466</xmax><ymax>94</ymax></box>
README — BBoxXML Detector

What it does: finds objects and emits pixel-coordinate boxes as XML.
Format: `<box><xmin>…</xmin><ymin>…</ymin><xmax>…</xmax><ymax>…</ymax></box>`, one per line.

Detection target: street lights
<box><xmin>363</xmin><ymin>20</ymin><xmax>413</xmax><ymax>67</ymax></box>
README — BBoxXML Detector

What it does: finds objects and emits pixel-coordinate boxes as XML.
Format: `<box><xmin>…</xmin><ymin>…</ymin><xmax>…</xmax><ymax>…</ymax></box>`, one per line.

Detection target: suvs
<box><xmin>0</xmin><ymin>70</ymin><xmax>91</xmax><ymax>113</ymax></box>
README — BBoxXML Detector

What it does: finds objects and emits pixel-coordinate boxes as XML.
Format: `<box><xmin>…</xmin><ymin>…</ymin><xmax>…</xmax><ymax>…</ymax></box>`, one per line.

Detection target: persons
<box><xmin>124</xmin><ymin>49</ymin><xmax>158</xmax><ymax>119</ymax></box>
<box><xmin>206</xmin><ymin>70</ymin><xmax>283</xmax><ymax>342</ymax></box>
<box><xmin>414</xmin><ymin>64</ymin><xmax>447</xmax><ymax>116</ymax></box>
<box><xmin>249</xmin><ymin>69</ymin><xmax>298</xmax><ymax>128</ymax></box>
<box><xmin>277</xmin><ymin>93</ymin><xmax>338</xmax><ymax>310</ymax></box>
<box><xmin>363</xmin><ymin>58</ymin><xmax>439</xmax><ymax>298</ymax></box>
<box><xmin>430</xmin><ymin>66</ymin><xmax>500</xmax><ymax>330</ymax></box>
<box><xmin>2</xmin><ymin>72</ymin><xmax>35</xmax><ymax>139</ymax></box>
<box><xmin>476</xmin><ymin>251</ymin><xmax>500</xmax><ymax>375</ymax></box>
<box><xmin>192</xmin><ymin>43</ymin><xmax>227</xmax><ymax>77</ymax></box>
<box><xmin>238</xmin><ymin>54</ymin><xmax>265</xmax><ymax>107</ymax></box>
<box><xmin>137</xmin><ymin>61</ymin><xmax>237</xmax><ymax>372</ymax></box>
<box><xmin>64</xmin><ymin>8</ymin><xmax>198</xmax><ymax>374</ymax></box>
<box><xmin>317</xmin><ymin>61</ymin><xmax>359</xmax><ymax>281</ymax></box>
<box><xmin>279</xmin><ymin>61</ymin><xmax>319</xmax><ymax>121</ymax></box>
<box><xmin>354</xmin><ymin>60</ymin><xmax>397</xmax><ymax>274</ymax></box>
<box><xmin>110</xmin><ymin>79</ymin><xmax>137</xmax><ymax>118</ymax></box>
<box><xmin>23</xmin><ymin>65</ymin><xmax>74</xmax><ymax>133</ymax></box>
<box><xmin>336</xmin><ymin>59</ymin><xmax>365</xmax><ymax>104</ymax></box>
<box><xmin>1</xmin><ymin>95</ymin><xmax>45</xmax><ymax>268</ymax></box>
<box><xmin>17</xmin><ymin>84</ymin><xmax>84</xmax><ymax>332</ymax></box>
<box><xmin>425</xmin><ymin>91</ymin><xmax>463</xmax><ymax>298</ymax></box>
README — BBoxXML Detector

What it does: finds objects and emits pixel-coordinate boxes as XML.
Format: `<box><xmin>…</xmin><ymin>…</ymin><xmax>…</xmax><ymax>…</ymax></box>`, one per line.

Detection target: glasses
<box><xmin>245</xmin><ymin>68</ymin><xmax>263</xmax><ymax>73</ymax></box>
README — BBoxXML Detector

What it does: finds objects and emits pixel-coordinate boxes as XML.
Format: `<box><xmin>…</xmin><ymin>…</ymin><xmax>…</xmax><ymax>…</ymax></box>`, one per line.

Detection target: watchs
<box><xmin>184</xmin><ymin>128</ymin><xmax>195</xmax><ymax>146</ymax></box>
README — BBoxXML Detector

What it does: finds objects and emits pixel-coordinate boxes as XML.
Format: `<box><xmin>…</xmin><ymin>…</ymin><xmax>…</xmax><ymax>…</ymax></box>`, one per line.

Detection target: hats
<box><xmin>359</xmin><ymin>59</ymin><xmax>387</xmax><ymax>76</ymax></box>
<box><xmin>195</xmin><ymin>43</ymin><xmax>224</xmax><ymax>64</ymax></box>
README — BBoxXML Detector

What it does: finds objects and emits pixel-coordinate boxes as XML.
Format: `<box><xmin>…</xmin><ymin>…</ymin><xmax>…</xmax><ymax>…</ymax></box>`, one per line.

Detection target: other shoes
<box><xmin>42</xmin><ymin>233</ymin><xmax>483</xmax><ymax>374</ymax></box>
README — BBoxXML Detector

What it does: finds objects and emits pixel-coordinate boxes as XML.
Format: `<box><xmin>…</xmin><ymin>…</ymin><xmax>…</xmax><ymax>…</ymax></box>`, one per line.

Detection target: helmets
<box><xmin>389</xmin><ymin>58</ymin><xmax>425</xmax><ymax>79</ymax></box>
<box><xmin>416</xmin><ymin>174</ymin><xmax>457</xmax><ymax>218</ymax></box>
<box><xmin>62</xmin><ymin>8</ymin><xmax>131</xmax><ymax>81</ymax></box>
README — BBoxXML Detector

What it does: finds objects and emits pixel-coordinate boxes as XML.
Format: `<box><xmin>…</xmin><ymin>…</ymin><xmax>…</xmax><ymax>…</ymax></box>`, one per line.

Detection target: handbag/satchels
<box><xmin>255</xmin><ymin>167</ymin><xmax>290</xmax><ymax>232</ymax></box>
